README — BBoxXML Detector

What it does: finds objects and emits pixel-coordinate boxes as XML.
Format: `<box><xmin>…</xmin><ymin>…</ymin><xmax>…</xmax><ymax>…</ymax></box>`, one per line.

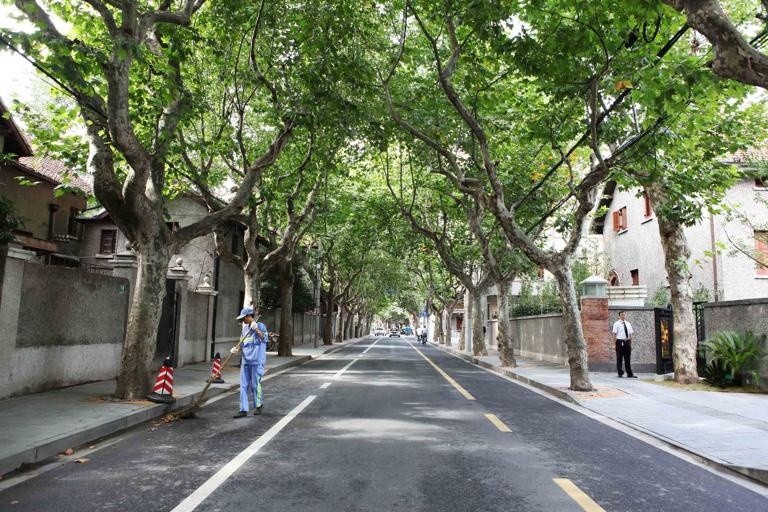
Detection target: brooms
<box><xmin>178</xmin><ymin>314</ymin><xmax>262</xmax><ymax>420</ymax></box>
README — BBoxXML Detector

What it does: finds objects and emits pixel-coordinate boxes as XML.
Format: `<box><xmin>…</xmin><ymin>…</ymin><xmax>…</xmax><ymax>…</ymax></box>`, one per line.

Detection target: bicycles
<box><xmin>417</xmin><ymin>333</ymin><xmax>428</xmax><ymax>345</ymax></box>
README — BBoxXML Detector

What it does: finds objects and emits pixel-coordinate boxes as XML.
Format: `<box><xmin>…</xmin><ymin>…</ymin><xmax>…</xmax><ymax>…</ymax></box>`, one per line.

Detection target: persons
<box><xmin>611</xmin><ymin>310</ymin><xmax>638</xmax><ymax>378</ymax></box>
<box><xmin>421</xmin><ymin>325</ymin><xmax>427</xmax><ymax>344</ymax></box>
<box><xmin>230</xmin><ymin>307</ymin><xmax>268</xmax><ymax>418</ymax></box>
<box><xmin>416</xmin><ymin>325</ymin><xmax>423</xmax><ymax>341</ymax></box>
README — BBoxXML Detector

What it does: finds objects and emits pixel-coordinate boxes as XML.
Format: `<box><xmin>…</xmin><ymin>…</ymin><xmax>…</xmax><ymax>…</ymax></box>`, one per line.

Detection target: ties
<box><xmin>623</xmin><ymin>322</ymin><xmax>628</xmax><ymax>338</ymax></box>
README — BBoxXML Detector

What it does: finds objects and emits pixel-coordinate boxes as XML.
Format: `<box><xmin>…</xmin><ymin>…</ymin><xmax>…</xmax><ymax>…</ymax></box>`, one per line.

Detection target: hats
<box><xmin>236</xmin><ymin>309</ymin><xmax>253</xmax><ymax>319</ymax></box>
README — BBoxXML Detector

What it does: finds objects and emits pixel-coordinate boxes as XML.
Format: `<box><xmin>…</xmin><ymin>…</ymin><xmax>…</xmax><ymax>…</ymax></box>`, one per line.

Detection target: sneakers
<box><xmin>254</xmin><ymin>405</ymin><xmax>263</xmax><ymax>415</ymax></box>
<box><xmin>619</xmin><ymin>375</ymin><xmax>621</xmax><ymax>377</ymax></box>
<box><xmin>632</xmin><ymin>376</ymin><xmax>637</xmax><ymax>378</ymax></box>
<box><xmin>233</xmin><ymin>411</ymin><xmax>246</xmax><ymax>418</ymax></box>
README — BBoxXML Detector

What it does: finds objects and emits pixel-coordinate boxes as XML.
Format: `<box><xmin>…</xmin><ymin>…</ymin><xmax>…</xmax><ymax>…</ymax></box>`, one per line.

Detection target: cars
<box><xmin>388</xmin><ymin>328</ymin><xmax>400</xmax><ymax>337</ymax></box>
<box><xmin>375</xmin><ymin>328</ymin><xmax>386</xmax><ymax>336</ymax></box>
<box><xmin>401</xmin><ymin>327</ymin><xmax>413</xmax><ymax>335</ymax></box>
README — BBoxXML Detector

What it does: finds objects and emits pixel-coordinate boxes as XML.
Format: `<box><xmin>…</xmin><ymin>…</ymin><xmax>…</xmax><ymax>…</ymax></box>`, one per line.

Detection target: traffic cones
<box><xmin>205</xmin><ymin>351</ymin><xmax>225</xmax><ymax>384</ymax></box>
<box><xmin>146</xmin><ymin>356</ymin><xmax>177</xmax><ymax>404</ymax></box>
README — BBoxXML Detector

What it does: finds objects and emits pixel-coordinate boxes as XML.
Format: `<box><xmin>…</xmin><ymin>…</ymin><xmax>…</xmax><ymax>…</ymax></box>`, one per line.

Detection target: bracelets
<box><xmin>256</xmin><ymin>331</ymin><xmax>262</xmax><ymax>334</ymax></box>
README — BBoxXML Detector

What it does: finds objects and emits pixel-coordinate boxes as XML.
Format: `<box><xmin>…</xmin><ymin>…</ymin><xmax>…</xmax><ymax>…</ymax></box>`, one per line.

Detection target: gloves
<box><xmin>230</xmin><ymin>347</ymin><xmax>239</xmax><ymax>354</ymax></box>
<box><xmin>251</xmin><ymin>322</ymin><xmax>258</xmax><ymax>331</ymax></box>
